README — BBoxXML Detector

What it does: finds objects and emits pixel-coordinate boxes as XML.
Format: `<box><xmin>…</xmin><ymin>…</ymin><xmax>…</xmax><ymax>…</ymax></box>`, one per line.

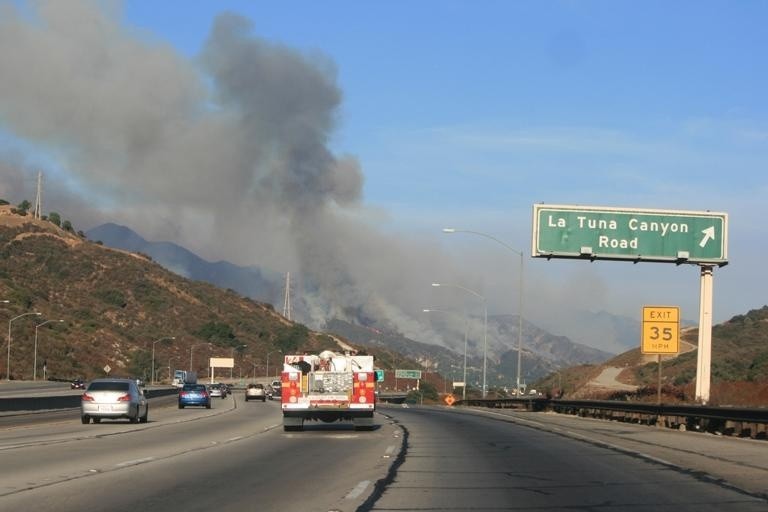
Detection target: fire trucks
<box><xmin>281</xmin><ymin>350</ymin><xmax>378</xmax><ymax>431</ymax></box>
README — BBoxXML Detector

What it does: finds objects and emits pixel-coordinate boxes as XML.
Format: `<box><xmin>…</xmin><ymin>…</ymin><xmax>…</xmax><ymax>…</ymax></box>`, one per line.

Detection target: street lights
<box><xmin>151</xmin><ymin>337</ymin><xmax>180</xmax><ymax>386</ymax></box>
<box><xmin>190</xmin><ymin>343</ymin><xmax>212</xmax><ymax>371</ymax></box>
<box><xmin>208</xmin><ymin>355</ymin><xmax>225</xmax><ymax>377</ymax></box>
<box><xmin>267</xmin><ymin>350</ymin><xmax>282</xmax><ymax>377</ymax></box>
<box><xmin>442</xmin><ymin>228</ymin><xmax>523</xmax><ymax>398</ymax></box>
<box><xmin>424</xmin><ymin>309</ymin><xmax>466</xmax><ymax>400</ymax></box>
<box><xmin>0</xmin><ymin>300</ymin><xmax>10</xmax><ymax>303</ymax></box>
<box><xmin>432</xmin><ymin>283</ymin><xmax>487</xmax><ymax>397</ymax></box>
<box><xmin>231</xmin><ymin>345</ymin><xmax>248</xmax><ymax>379</ymax></box>
<box><xmin>34</xmin><ymin>320</ymin><xmax>65</xmax><ymax>381</ymax></box>
<box><xmin>7</xmin><ymin>313</ymin><xmax>42</xmax><ymax>381</ymax></box>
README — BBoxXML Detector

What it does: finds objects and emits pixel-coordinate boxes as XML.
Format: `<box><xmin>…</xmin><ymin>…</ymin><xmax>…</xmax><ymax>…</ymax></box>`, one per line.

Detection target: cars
<box><xmin>81</xmin><ymin>379</ymin><xmax>148</xmax><ymax>424</ymax></box>
<box><xmin>178</xmin><ymin>384</ymin><xmax>212</xmax><ymax>408</ymax></box>
<box><xmin>71</xmin><ymin>379</ymin><xmax>84</xmax><ymax>389</ymax></box>
<box><xmin>208</xmin><ymin>383</ymin><xmax>233</xmax><ymax>399</ymax></box>
<box><xmin>245</xmin><ymin>380</ymin><xmax>281</xmax><ymax>402</ymax></box>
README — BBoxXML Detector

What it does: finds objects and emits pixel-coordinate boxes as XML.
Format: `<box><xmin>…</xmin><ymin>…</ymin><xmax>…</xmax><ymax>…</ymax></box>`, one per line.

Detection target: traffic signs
<box><xmin>395</xmin><ymin>369</ymin><xmax>421</xmax><ymax>379</ymax></box>
<box><xmin>376</xmin><ymin>370</ymin><xmax>384</xmax><ymax>382</ymax></box>
<box><xmin>531</xmin><ymin>204</ymin><xmax>728</xmax><ymax>268</ymax></box>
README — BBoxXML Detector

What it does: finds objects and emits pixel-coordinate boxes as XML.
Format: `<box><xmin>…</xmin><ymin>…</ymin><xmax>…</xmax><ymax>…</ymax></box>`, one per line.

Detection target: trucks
<box><xmin>174</xmin><ymin>370</ymin><xmax>197</xmax><ymax>384</ymax></box>
